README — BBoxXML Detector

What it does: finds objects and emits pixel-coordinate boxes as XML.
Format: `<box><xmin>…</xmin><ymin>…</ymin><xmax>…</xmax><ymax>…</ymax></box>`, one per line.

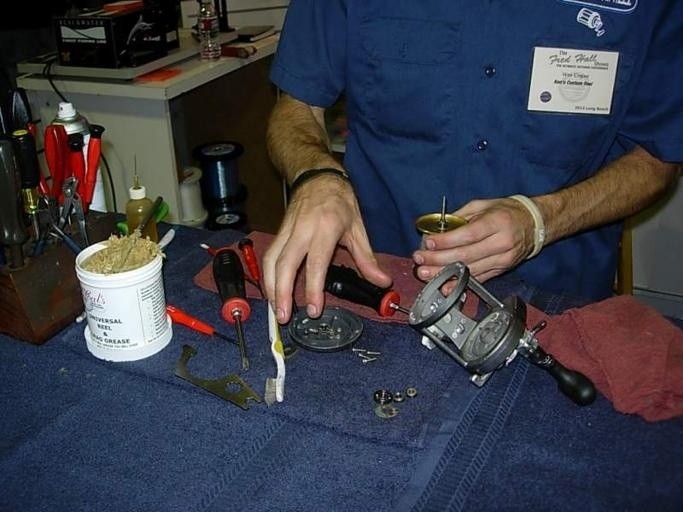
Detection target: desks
<box><xmin>0</xmin><ymin>211</ymin><xmax>683</xmax><ymax>511</ymax></box>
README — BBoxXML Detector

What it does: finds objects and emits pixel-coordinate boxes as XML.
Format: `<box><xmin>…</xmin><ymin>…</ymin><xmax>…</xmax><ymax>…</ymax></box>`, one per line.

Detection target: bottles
<box><xmin>50</xmin><ymin>102</ymin><xmax>109</xmax><ymax>217</ymax></box>
<box><xmin>197</xmin><ymin>8</ymin><xmax>221</xmax><ymax>62</ymax></box>
<box><xmin>126</xmin><ymin>186</ymin><xmax>160</xmax><ymax>246</ymax></box>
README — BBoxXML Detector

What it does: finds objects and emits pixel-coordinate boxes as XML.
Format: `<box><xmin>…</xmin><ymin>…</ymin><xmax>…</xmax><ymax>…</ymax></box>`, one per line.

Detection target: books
<box><xmin>228</xmin><ymin>25</ymin><xmax>281</xmax><ymax>50</ymax></box>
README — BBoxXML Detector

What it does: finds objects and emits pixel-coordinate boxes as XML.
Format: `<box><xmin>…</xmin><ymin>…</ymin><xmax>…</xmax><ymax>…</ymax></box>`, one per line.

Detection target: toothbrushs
<box><xmin>264</xmin><ymin>300</ymin><xmax>287</xmax><ymax>406</ymax></box>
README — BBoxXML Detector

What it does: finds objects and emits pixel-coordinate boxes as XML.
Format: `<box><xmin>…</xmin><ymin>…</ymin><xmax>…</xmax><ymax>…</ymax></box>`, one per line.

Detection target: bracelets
<box><xmin>292</xmin><ymin>168</ymin><xmax>349</xmax><ymax>190</ymax></box>
<box><xmin>509</xmin><ymin>194</ymin><xmax>545</xmax><ymax>260</ymax></box>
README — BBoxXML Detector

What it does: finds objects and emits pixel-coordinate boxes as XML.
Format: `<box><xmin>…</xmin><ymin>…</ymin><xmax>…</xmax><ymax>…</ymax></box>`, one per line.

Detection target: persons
<box><xmin>261</xmin><ymin>0</ymin><xmax>682</xmax><ymax>324</ymax></box>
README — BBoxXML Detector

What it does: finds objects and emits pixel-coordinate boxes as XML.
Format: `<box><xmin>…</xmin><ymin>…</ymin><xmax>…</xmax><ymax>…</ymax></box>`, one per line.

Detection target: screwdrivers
<box><xmin>212</xmin><ymin>251</ymin><xmax>252</xmax><ymax>370</ymax></box>
<box><xmin>324</xmin><ymin>265</ymin><xmax>410</xmax><ymax>319</ymax></box>
<box><xmin>167</xmin><ymin>305</ymin><xmax>240</xmax><ymax>347</ymax></box>
<box><xmin>11</xmin><ymin>130</ymin><xmax>40</xmax><ymax>241</ymax></box>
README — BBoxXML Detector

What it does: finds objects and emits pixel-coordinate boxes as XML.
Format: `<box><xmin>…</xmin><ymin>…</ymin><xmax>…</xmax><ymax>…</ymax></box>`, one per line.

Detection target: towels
<box><xmin>524</xmin><ymin>291</ymin><xmax>683</xmax><ymax>424</ymax></box>
<box><xmin>192</xmin><ymin>225</ymin><xmax>480</xmax><ymax>326</ymax></box>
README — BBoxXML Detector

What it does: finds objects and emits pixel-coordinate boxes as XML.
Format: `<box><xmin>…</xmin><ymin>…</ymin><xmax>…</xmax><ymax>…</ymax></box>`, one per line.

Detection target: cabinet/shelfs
<box><xmin>14</xmin><ymin>30</ymin><xmax>286</xmax><ymax>234</ymax></box>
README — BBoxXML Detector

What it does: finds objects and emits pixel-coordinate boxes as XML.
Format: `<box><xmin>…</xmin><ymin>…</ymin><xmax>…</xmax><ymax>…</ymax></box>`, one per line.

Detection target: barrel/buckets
<box><xmin>73</xmin><ymin>237</ymin><xmax>173</xmax><ymax>362</ymax></box>
